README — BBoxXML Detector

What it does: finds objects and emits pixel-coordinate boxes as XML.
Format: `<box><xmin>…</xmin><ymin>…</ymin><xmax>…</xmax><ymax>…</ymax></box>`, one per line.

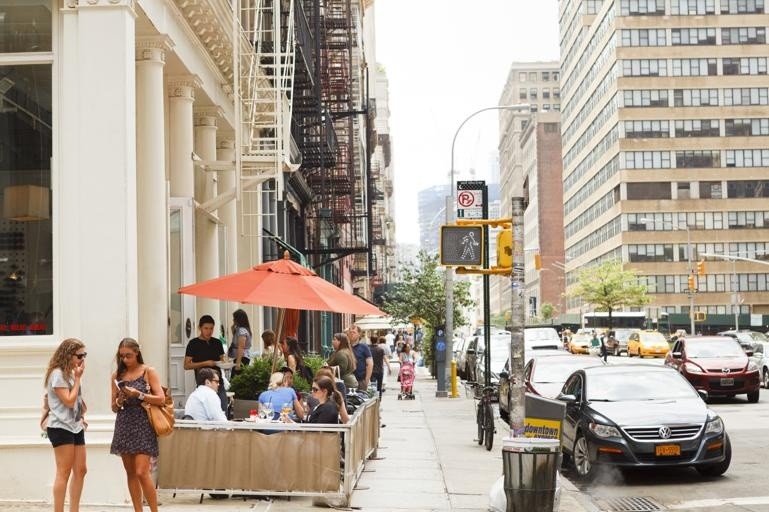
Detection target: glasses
<box><xmin>72</xmin><ymin>352</ymin><xmax>87</xmax><ymax>359</ymax></box>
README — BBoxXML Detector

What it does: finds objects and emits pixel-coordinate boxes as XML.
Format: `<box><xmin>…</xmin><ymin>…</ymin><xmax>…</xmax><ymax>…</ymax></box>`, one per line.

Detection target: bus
<box><xmin>582</xmin><ymin>311</ymin><xmax>647</xmax><ymax>336</ymax></box>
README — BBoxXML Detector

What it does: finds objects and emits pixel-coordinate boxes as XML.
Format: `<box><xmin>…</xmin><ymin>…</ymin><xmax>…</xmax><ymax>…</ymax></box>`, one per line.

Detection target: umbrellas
<box><xmin>177</xmin><ymin>249</ymin><xmax>389</xmax><ymax>376</ymax></box>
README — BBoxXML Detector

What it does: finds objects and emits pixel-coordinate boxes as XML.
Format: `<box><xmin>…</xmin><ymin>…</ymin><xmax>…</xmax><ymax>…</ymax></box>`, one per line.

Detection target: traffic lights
<box><xmin>688</xmin><ymin>275</ymin><xmax>695</xmax><ymax>289</ymax></box>
<box><xmin>694</xmin><ymin>312</ymin><xmax>705</xmax><ymax>321</ymax></box>
<box><xmin>696</xmin><ymin>260</ymin><xmax>705</xmax><ymax>277</ymax></box>
<box><xmin>441</xmin><ymin>225</ymin><xmax>482</xmax><ymax>265</ymax></box>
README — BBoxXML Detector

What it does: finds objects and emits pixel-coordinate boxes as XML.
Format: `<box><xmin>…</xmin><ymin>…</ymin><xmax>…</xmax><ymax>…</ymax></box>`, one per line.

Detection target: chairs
<box><xmin>306</xmin><ymin>414</ymin><xmax>312</xmax><ymax>423</ymax></box>
<box><xmin>155</xmin><ymin>415</ymin><xmax>204</xmax><ymax>504</ymax></box>
<box><xmin>225</xmin><ymin>399</ymin><xmax>259</xmax><ymax>421</ymax></box>
<box><xmin>343</xmin><ymin>389</ymin><xmax>371</xmax><ymax>415</ymax></box>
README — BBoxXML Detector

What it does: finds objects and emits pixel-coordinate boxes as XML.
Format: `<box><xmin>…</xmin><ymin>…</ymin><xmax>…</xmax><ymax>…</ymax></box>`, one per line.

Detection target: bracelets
<box><xmin>137</xmin><ymin>392</ymin><xmax>144</xmax><ymax>401</ymax></box>
<box><xmin>115</xmin><ymin>398</ymin><xmax>123</xmax><ymax>408</ymax></box>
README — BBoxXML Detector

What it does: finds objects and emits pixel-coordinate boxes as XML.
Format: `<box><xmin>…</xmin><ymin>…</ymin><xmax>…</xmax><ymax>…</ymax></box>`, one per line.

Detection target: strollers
<box><xmin>397</xmin><ymin>362</ymin><xmax>415</xmax><ymax>401</ymax></box>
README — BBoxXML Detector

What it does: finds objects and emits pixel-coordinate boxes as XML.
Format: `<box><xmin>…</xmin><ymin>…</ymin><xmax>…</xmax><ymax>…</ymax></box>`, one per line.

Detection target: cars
<box><xmin>451</xmin><ymin>326</ymin><xmax>566</xmax><ymax>403</ymax></box>
<box><xmin>716</xmin><ymin>329</ymin><xmax>769</xmax><ymax>389</ymax></box>
<box><xmin>553</xmin><ymin>364</ymin><xmax>732</xmax><ymax>480</ymax></box>
<box><xmin>523</xmin><ymin>354</ymin><xmax>608</xmax><ymax>400</ymax></box>
<box><xmin>567</xmin><ymin>334</ymin><xmax>592</xmax><ymax>354</ymax></box>
<box><xmin>497</xmin><ymin>349</ymin><xmax>571</xmax><ymax>420</ymax></box>
<box><xmin>600</xmin><ymin>329</ymin><xmax>631</xmax><ymax>356</ymax></box>
<box><xmin>664</xmin><ymin>335</ymin><xmax>761</xmax><ymax>403</ymax></box>
<box><xmin>625</xmin><ymin>332</ymin><xmax>670</xmax><ymax>358</ymax></box>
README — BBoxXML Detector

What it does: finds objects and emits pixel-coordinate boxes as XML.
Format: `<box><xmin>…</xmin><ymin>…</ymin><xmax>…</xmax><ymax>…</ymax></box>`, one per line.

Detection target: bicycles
<box><xmin>465</xmin><ymin>381</ymin><xmax>502</xmax><ymax>451</ymax></box>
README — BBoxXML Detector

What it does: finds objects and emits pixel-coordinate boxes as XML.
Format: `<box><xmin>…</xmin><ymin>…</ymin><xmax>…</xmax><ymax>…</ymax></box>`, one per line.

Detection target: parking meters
<box><xmin>434</xmin><ymin>326</ymin><xmax>446</xmax><ymax>391</ymax></box>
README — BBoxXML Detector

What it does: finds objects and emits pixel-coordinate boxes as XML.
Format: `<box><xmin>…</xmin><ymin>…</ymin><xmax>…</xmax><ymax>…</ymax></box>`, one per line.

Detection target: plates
<box><xmin>245</xmin><ymin>418</ymin><xmax>256</xmax><ymax>422</ymax></box>
<box><xmin>214</xmin><ymin>360</ymin><xmax>235</xmax><ymax>370</ymax></box>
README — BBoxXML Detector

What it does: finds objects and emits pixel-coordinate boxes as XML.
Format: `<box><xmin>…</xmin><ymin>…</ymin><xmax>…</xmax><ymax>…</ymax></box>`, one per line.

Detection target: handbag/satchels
<box><xmin>141</xmin><ymin>386</ymin><xmax>175</xmax><ymax>437</ymax></box>
<box><xmin>41</xmin><ymin>394</ymin><xmax>87</xmax><ymax>432</ymax></box>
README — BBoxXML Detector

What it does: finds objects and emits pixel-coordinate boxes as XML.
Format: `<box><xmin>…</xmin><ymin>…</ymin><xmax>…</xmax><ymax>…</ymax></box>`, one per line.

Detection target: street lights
<box><xmin>639</xmin><ymin>217</ymin><xmax>696</xmax><ymax>335</ymax></box>
<box><xmin>444</xmin><ymin>101</ymin><xmax>531</xmax><ymax>388</ymax></box>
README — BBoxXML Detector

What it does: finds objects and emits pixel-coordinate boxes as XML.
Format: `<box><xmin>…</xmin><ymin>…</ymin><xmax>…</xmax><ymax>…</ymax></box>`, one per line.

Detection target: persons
<box><xmin>184</xmin><ymin>315</ymin><xmax>228</xmax><ymax>412</ymax></box>
<box><xmin>262</xmin><ymin>329</ymin><xmax>284</xmax><ymax>360</ymax></box>
<box><xmin>327</xmin><ymin>333</ymin><xmax>359</xmax><ymax>392</ymax></box>
<box><xmin>587</xmin><ymin>325</ymin><xmax>617</xmax><ymax>361</ymax></box>
<box><xmin>348</xmin><ymin>325</ymin><xmax>374</xmax><ymax>391</ymax></box>
<box><xmin>561</xmin><ymin>331</ymin><xmax>571</xmax><ymax>351</ymax></box>
<box><xmin>40</xmin><ymin>338</ymin><xmax>86</xmax><ymax>512</ymax></box>
<box><xmin>185</xmin><ymin>368</ymin><xmax>229</xmax><ymax>499</ymax></box>
<box><xmin>111</xmin><ymin>338</ymin><xmax>166</xmax><ymax>512</ymax></box>
<box><xmin>229</xmin><ymin>310</ymin><xmax>252</xmax><ymax>383</ymax></box>
<box><xmin>258</xmin><ymin>367</ymin><xmax>349</xmax><ymax>435</ymax></box>
<box><xmin>366</xmin><ymin>330</ymin><xmax>418</xmax><ymax>400</ymax></box>
<box><xmin>282</xmin><ymin>336</ymin><xmax>305</xmax><ymax>375</ymax></box>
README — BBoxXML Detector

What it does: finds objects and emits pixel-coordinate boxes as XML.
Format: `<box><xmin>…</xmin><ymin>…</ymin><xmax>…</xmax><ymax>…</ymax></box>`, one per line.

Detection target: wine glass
<box><xmin>347</xmin><ymin>387</ymin><xmax>357</xmax><ymax>396</ymax></box>
<box><xmin>281</xmin><ymin>403</ymin><xmax>293</xmax><ymax>423</ymax></box>
<box><xmin>258</xmin><ymin>408</ymin><xmax>265</xmax><ymax>422</ymax></box>
<box><xmin>262</xmin><ymin>402</ymin><xmax>276</xmax><ymax>424</ymax></box>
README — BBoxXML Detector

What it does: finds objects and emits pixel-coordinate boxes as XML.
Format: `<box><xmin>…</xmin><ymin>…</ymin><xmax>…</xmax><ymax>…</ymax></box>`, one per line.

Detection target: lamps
<box><xmin>2</xmin><ymin>185</ymin><xmax>49</xmax><ymax>221</ymax></box>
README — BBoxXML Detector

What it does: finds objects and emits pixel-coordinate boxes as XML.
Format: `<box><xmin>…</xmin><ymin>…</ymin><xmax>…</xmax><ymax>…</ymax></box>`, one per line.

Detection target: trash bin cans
<box><xmin>501</xmin><ymin>438</ymin><xmax>562</xmax><ymax>512</ymax></box>
